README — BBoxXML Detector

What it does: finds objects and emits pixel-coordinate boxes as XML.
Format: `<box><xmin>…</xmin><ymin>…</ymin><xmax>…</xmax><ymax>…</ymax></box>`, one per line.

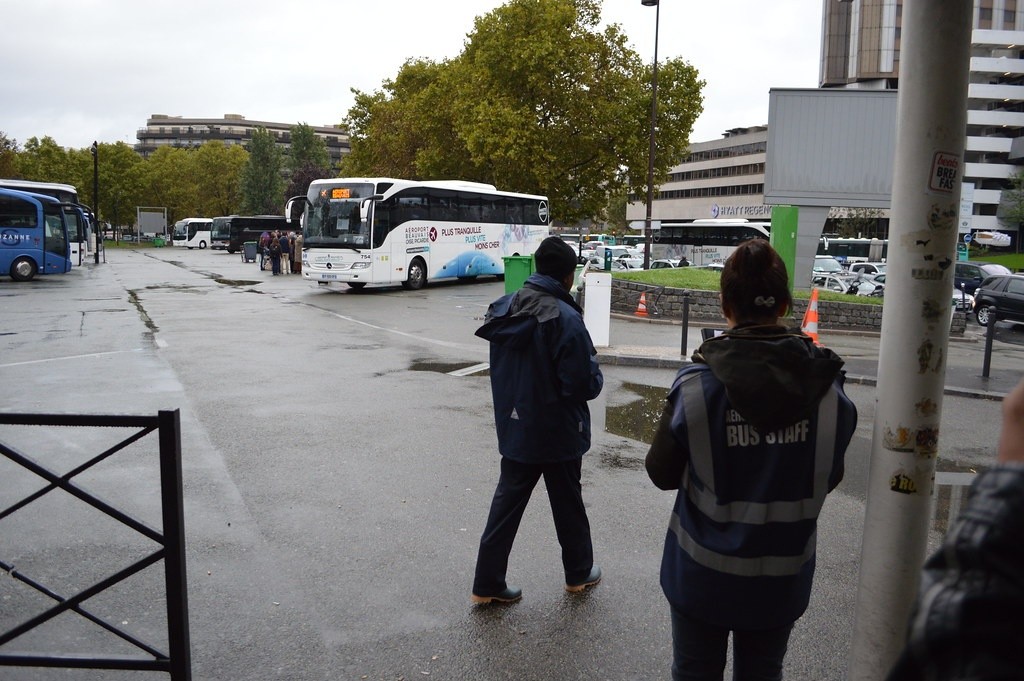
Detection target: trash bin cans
<box><xmin>570</xmin><ymin>262</ymin><xmax>588</xmax><ymax>305</ymax></box>
<box><xmin>151</xmin><ymin>237</ymin><xmax>165</xmax><ymax>247</ymax></box>
<box><xmin>500</xmin><ymin>255</ymin><xmax>531</xmax><ymax>297</ymax></box>
<box><xmin>242</xmin><ymin>241</ymin><xmax>257</xmax><ymax>263</ymax></box>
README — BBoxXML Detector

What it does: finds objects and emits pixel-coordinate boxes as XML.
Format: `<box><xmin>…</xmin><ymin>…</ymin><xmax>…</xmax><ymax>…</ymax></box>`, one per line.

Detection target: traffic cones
<box><xmin>799</xmin><ymin>288</ymin><xmax>825</xmax><ymax>349</ymax></box>
<box><xmin>633</xmin><ymin>292</ymin><xmax>649</xmax><ymax>317</ymax></box>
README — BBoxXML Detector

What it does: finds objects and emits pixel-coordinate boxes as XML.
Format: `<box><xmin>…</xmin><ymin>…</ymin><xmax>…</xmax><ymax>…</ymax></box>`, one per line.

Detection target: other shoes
<box><xmin>292</xmin><ymin>271</ymin><xmax>298</xmax><ymax>274</ymax></box>
<box><xmin>273</xmin><ymin>272</ymin><xmax>278</xmax><ymax>275</ymax></box>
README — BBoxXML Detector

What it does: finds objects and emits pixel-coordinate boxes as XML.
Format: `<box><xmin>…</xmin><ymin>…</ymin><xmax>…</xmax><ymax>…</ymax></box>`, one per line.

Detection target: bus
<box><xmin>817</xmin><ymin>237</ymin><xmax>889</xmax><ymax>268</ymax></box>
<box><xmin>205</xmin><ymin>214</ymin><xmax>300</xmax><ymax>253</ymax></box>
<box><xmin>0</xmin><ymin>188</ymin><xmax>72</xmax><ymax>281</ymax></box>
<box><xmin>285</xmin><ymin>176</ymin><xmax>549</xmax><ymax>288</ymax></box>
<box><xmin>621</xmin><ymin>235</ymin><xmax>652</xmax><ymax>247</ymax></box>
<box><xmin>560</xmin><ymin>234</ymin><xmax>616</xmax><ymax>247</ymax></box>
<box><xmin>652</xmin><ymin>218</ymin><xmax>772</xmax><ymax>265</ymax></box>
<box><xmin>0</xmin><ymin>179</ymin><xmax>108</xmax><ymax>266</ymax></box>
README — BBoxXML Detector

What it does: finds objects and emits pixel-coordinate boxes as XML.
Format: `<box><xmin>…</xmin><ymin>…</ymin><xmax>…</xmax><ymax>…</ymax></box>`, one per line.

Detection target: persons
<box><xmin>259</xmin><ymin>230</ymin><xmax>302</xmax><ymax>275</ymax></box>
<box><xmin>887</xmin><ymin>380</ymin><xmax>1024</xmax><ymax>681</ymax></box>
<box><xmin>472</xmin><ymin>237</ymin><xmax>604</xmax><ymax>603</ymax></box>
<box><xmin>646</xmin><ymin>242</ymin><xmax>858</xmax><ymax>681</ymax></box>
<box><xmin>678</xmin><ymin>256</ymin><xmax>689</xmax><ymax>267</ymax></box>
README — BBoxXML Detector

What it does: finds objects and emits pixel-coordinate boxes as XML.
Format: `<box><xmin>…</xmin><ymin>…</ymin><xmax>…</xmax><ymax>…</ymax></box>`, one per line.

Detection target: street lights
<box><xmin>638</xmin><ymin>0</ymin><xmax>658</xmax><ymax>268</ymax></box>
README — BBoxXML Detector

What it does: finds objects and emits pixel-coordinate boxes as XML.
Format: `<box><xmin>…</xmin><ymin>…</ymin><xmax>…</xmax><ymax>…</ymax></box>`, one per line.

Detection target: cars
<box><xmin>951</xmin><ymin>288</ymin><xmax>976</xmax><ymax>316</ymax></box>
<box><xmin>974</xmin><ymin>274</ymin><xmax>1024</xmax><ymax>327</ymax></box>
<box><xmin>169</xmin><ymin>217</ymin><xmax>215</xmax><ymax>249</ymax></box>
<box><xmin>651</xmin><ymin>259</ymin><xmax>694</xmax><ymax>269</ymax></box>
<box><xmin>811</xmin><ymin>255</ymin><xmax>886</xmax><ymax>299</ymax></box>
<box><xmin>566</xmin><ymin>241</ymin><xmax>652</xmax><ymax>271</ymax></box>
<box><xmin>954</xmin><ymin>260</ymin><xmax>1013</xmax><ymax>297</ymax></box>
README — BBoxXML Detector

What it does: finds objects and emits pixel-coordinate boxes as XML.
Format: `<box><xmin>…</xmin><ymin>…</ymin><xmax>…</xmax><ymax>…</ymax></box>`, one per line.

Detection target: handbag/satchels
<box><xmin>267</xmin><ymin>249</ymin><xmax>281</xmax><ymax>258</ymax></box>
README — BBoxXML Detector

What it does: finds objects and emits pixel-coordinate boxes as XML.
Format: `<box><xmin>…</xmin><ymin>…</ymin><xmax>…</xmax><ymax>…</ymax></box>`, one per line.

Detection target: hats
<box><xmin>533</xmin><ymin>238</ymin><xmax>578</xmax><ymax>275</ymax></box>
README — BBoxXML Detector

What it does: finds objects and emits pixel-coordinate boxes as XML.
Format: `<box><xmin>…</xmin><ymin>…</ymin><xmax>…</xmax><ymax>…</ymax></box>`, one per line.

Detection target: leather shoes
<box><xmin>563</xmin><ymin>566</ymin><xmax>603</xmax><ymax>592</ymax></box>
<box><xmin>470</xmin><ymin>587</ymin><xmax>522</xmax><ymax>604</ymax></box>
